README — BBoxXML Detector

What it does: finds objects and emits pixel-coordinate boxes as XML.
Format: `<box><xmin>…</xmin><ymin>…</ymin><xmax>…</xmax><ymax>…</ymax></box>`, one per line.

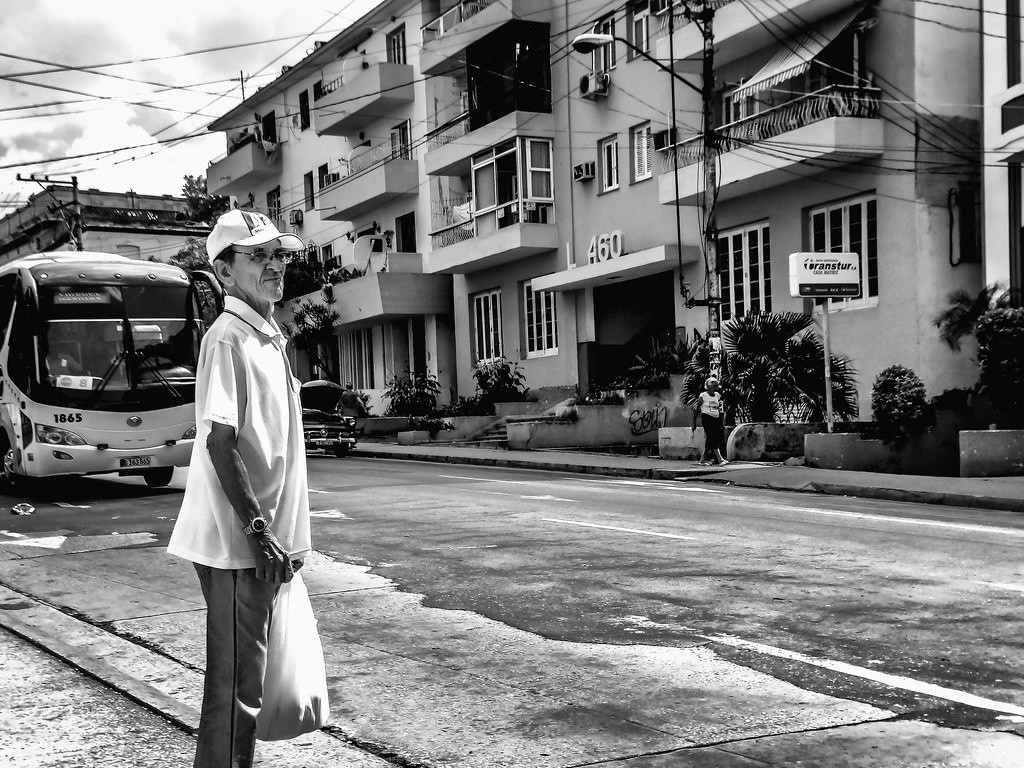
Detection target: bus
<box><xmin>0</xmin><ymin>251</ymin><xmax>225</xmax><ymax>496</ymax></box>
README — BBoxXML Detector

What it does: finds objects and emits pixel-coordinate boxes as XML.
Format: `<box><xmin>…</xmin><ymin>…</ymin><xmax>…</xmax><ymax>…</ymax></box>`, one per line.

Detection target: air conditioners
<box><xmin>578</xmin><ymin>71</ymin><xmax>603</xmax><ymax>98</ymax></box>
<box><xmin>573</xmin><ymin>162</ymin><xmax>594</xmax><ymax>182</ymax></box>
<box><xmin>289</xmin><ymin>210</ymin><xmax>303</xmax><ymax>224</ymax></box>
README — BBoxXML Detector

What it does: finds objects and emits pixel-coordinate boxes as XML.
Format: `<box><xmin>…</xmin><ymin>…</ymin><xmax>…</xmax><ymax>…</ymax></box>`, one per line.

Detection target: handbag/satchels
<box><xmin>254</xmin><ymin>571</ymin><xmax>329</xmax><ymax>741</ymax></box>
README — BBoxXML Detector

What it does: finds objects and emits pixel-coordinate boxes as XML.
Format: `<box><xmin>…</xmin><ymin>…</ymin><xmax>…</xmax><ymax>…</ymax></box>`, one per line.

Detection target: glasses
<box><xmin>234</xmin><ymin>251</ymin><xmax>295</xmax><ymax>264</ymax></box>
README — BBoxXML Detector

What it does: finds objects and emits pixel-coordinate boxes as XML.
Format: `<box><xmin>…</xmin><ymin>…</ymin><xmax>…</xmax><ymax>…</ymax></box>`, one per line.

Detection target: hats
<box><xmin>206</xmin><ymin>209</ymin><xmax>304</xmax><ymax>266</ymax></box>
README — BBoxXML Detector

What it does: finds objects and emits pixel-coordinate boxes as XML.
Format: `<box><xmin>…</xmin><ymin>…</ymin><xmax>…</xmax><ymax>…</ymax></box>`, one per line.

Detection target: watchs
<box><xmin>243</xmin><ymin>517</ymin><xmax>268</xmax><ymax>535</ymax></box>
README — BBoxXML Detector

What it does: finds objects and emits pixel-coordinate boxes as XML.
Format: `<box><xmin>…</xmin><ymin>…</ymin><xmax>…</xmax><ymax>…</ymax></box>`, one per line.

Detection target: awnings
<box><xmin>733</xmin><ymin>4</ymin><xmax>868</xmax><ymax>104</ymax></box>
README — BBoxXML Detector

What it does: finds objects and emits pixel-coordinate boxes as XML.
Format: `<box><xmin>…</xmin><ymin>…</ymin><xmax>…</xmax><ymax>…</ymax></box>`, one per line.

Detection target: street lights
<box><xmin>572</xmin><ymin>32</ymin><xmax>726</xmax><ymax>424</ymax></box>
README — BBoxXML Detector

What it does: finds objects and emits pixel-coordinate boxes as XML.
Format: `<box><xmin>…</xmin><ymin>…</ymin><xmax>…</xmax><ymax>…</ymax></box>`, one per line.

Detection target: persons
<box><xmin>46</xmin><ymin>338</ymin><xmax>80</xmax><ymax>386</ymax></box>
<box><xmin>692</xmin><ymin>377</ymin><xmax>730</xmax><ymax>466</ymax></box>
<box><xmin>335</xmin><ymin>381</ymin><xmax>367</xmax><ymax>448</ymax></box>
<box><xmin>167</xmin><ymin>211</ymin><xmax>312</xmax><ymax>768</ymax></box>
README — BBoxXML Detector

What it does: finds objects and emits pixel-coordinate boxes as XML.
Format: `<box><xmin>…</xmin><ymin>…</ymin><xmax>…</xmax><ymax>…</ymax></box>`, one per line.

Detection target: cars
<box><xmin>301</xmin><ymin>379</ymin><xmax>358</xmax><ymax>458</ymax></box>
